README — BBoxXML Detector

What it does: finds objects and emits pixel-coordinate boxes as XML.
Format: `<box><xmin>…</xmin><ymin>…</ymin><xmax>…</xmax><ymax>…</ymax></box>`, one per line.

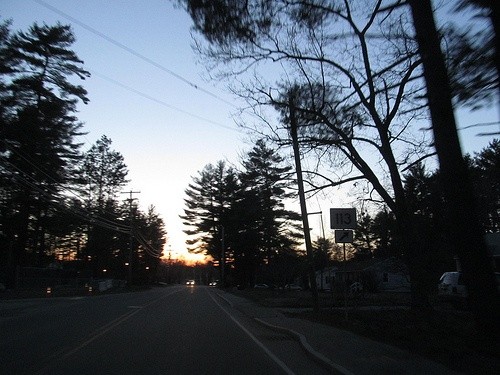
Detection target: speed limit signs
<box><xmin>330</xmin><ymin>208</ymin><xmax>356</xmax><ymax>229</ymax></box>
